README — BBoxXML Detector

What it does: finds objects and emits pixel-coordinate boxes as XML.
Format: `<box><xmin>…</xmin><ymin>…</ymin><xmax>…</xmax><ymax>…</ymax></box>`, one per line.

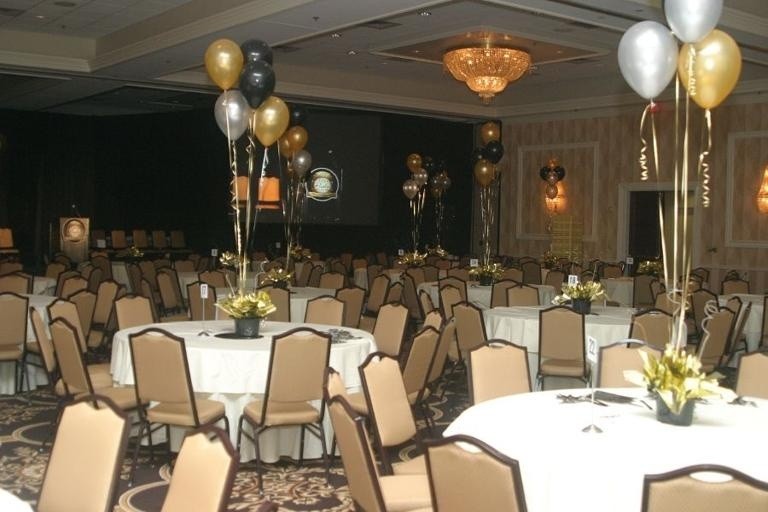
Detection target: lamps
<box><xmin>440</xmin><ymin>41</ymin><xmax>533</xmax><ymax>110</ymax></box>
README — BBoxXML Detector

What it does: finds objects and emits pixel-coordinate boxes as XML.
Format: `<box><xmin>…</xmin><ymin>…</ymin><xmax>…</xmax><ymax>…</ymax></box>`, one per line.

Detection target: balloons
<box><xmin>676</xmin><ymin>29</ymin><xmax>743</xmax><ymax>112</ymax></box>
<box><xmin>471</xmin><ymin>120</ymin><xmax>506</xmax><ymax>192</ymax></box>
<box><xmin>539</xmin><ymin>154</ymin><xmax>565</xmax><ymax>201</ymax></box>
<box><xmin>401</xmin><ymin>122</ymin><xmax>451</xmax><ymax>199</ymax></box>
<box><xmin>202</xmin><ymin>38</ymin><xmax>313</xmax><ymax>178</ymax></box>
<box><xmin>664</xmin><ymin>0</ymin><xmax>725</xmax><ymax>47</ymax></box>
<box><xmin>617</xmin><ymin>19</ymin><xmax>679</xmax><ymax>103</ymax></box>
